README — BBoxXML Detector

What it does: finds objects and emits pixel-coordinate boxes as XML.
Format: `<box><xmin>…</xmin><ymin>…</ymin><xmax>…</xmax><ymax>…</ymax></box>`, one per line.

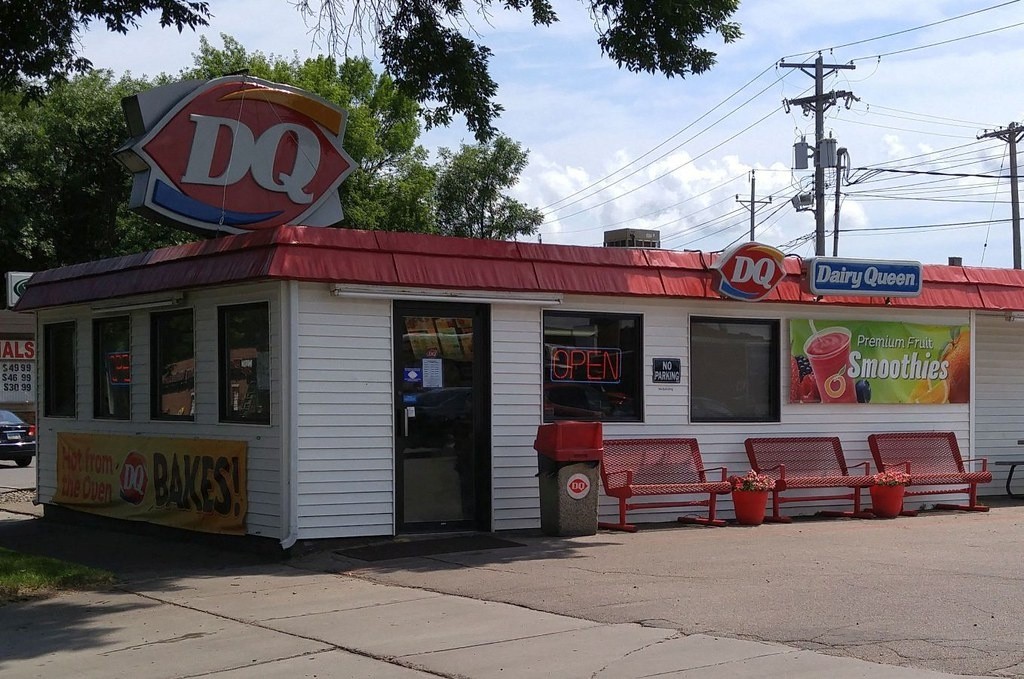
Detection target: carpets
<box><xmin>332</xmin><ymin>534</ymin><xmax>528</xmax><ymax>563</ymax></box>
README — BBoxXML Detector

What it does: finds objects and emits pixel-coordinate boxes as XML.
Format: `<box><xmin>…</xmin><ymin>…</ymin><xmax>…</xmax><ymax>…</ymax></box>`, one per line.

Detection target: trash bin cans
<box><xmin>533</xmin><ymin>421</ymin><xmax>603</xmax><ymax>536</ymax></box>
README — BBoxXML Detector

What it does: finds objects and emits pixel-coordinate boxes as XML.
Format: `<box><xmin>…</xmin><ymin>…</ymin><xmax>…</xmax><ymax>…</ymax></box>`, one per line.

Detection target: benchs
<box><xmin>744</xmin><ymin>436</ymin><xmax>876</xmax><ymax>523</ymax></box>
<box><xmin>995</xmin><ymin>460</ymin><xmax>1024</xmax><ymax>500</ymax></box>
<box><xmin>864</xmin><ymin>432</ymin><xmax>993</xmax><ymax>516</ymax></box>
<box><xmin>597</xmin><ymin>438</ymin><xmax>732</xmax><ymax>533</ymax></box>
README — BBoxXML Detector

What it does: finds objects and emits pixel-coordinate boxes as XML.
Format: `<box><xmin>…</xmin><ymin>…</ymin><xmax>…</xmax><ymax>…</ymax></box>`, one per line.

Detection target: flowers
<box><xmin>728</xmin><ymin>468</ymin><xmax>776</xmax><ymax>492</ymax></box>
<box><xmin>874</xmin><ymin>469</ymin><xmax>911</xmax><ymax>486</ymax></box>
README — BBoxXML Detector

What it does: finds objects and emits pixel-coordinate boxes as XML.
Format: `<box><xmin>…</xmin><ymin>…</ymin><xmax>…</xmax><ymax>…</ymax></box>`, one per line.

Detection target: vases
<box><xmin>869</xmin><ymin>484</ymin><xmax>905</xmax><ymax>518</ymax></box>
<box><xmin>732</xmin><ymin>490</ymin><xmax>769</xmax><ymax>526</ymax></box>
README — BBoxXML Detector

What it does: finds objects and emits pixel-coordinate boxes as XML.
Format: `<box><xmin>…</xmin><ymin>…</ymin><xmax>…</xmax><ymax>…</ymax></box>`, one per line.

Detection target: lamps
<box><xmin>329</xmin><ymin>283</ymin><xmax>564</xmax><ymax>308</ymax></box>
<box><xmin>91</xmin><ymin>291</ymin><xmax>185</xmax><ymax>314</ymax></box>
<box><xmin>1004</xmin><ymin>311</ymin><xmax>1024</xmax><ymax>321</ymax></box>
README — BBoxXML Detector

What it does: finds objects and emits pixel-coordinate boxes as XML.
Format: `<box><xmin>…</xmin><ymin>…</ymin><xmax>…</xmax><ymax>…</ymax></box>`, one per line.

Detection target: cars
<box><xmin>0</xmin><ymin>411</ymin><xmax>36</xmax><ymax>467</ymax></box>
<box><xmin>402</xmin><ymin>384</ymin><xmax>641</xmax><ymax>450</ymax></box>
